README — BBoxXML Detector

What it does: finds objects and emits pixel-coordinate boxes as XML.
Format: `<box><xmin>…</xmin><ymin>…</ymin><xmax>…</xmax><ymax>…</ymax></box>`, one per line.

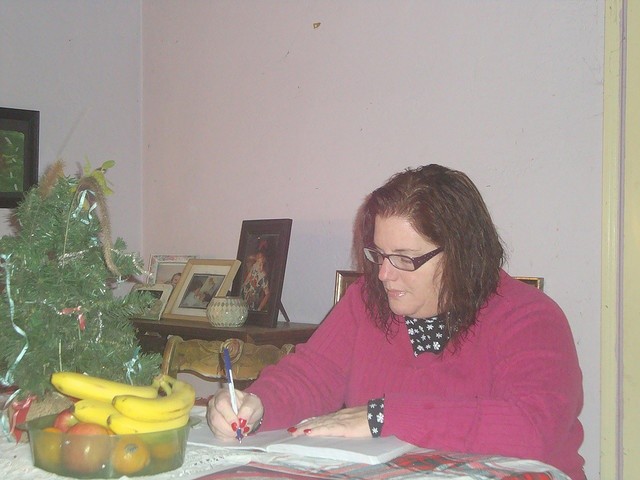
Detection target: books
<box><xmin>185</xmin><ymin>414</ymin><xmax>416</xmax><ymax>466</ymax></box>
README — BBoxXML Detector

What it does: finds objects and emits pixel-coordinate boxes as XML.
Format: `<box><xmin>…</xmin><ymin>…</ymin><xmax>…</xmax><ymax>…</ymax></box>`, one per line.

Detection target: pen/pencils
<box><xmin>224</xmin><ymin>349</ymin><xmax>242</xmax><ymax>444</ymax></box>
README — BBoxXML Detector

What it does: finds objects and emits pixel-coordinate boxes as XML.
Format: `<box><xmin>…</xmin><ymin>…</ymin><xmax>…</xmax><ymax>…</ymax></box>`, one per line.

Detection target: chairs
<box><xmin>158</xmin><ymin>334</ymin><xmax>295</xmax><ymax>399</ymax></box>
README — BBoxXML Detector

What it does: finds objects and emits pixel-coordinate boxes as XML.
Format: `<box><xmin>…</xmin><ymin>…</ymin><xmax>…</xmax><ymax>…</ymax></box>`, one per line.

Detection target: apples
<box><xmin>55</xmin><ymin>410</ymin><xmax>78</xmax><ymax>430</ymax></box>
<box><xmin>63</xmin><ymin>422</ymin><xmax>109</xmax><ymax>470</ymax></box>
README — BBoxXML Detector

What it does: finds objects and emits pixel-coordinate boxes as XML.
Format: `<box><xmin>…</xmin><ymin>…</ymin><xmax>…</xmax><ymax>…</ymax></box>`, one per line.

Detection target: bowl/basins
<box><xmin>16</xmin><ymin>411</ymin><xmax>202</xmax><ymax>479</ymax></box>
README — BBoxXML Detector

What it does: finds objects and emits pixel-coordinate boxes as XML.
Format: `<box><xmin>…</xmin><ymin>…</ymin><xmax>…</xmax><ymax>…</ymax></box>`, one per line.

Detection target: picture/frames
<box><xmin>162</xmin><ymin>257</ymin><xmax>241</xmax><ymax>322</ymax></box>
<box><xmin>516</xmin><ymin>276</ymin><xmax>543</xmax><ymax>291</ymax></box>
<box><xmin>123</xmin><ymin>283</ymin><xmax>170</xmax><ymax>322</ymax></box>
<box><xmin>0</xmin><ymin>107</ymin><xmax>42</xmax><ymax>208</ymax></box>
<box><xmin>333</xmin><ymin>270</ymin><xmax>369</xmax><ymax>306</ymax></box>
<box><xmin>146</xmin><ymin>254</ymin><xmax>196</xmax><ymax>286</ymax></box>
<box><xmin>231</xmin><ymin>219</ymin><xmax>296</xmax><ymax>329</ymax></box>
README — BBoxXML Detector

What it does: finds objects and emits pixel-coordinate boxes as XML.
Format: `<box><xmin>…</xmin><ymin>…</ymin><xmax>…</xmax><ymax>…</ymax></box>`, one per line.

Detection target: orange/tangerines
<box><xmin>36</xmin><ymin>428</ymin><xmax>63</xmax><ymax>465</ymax></box>
<box><xmin>147</xmin><ymin>433</ymin><xmax>179</xmax><ymax>458</ymax></box>
<box><xmin>109</xmin><ymin>433</ymin><xmax>150</xmax><ymax>474</ymax></box>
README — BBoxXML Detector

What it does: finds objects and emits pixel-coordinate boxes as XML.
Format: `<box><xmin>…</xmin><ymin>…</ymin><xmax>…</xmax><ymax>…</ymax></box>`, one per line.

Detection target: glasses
<box><xmin>363</xmin><ymin>242</ymin><xmax>443</xmax><ymax>272</ymax></box>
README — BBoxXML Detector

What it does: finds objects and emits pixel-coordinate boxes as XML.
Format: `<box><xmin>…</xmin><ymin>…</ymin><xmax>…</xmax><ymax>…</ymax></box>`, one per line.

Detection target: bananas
<box><xmin>50</xmin><ymin>372</ymin><xmax>195</xmax><ymax>433</ymax></box>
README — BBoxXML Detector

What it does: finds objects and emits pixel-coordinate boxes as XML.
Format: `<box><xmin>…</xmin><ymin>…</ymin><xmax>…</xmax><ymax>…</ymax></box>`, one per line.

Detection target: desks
<box><xmin>191</xmin><ymin>396</ymin><xmax>569</xmax><ymax>480</ymax></box>
<box><xmin>125</xmin><ymin>317</ymin><xmax>319</xmax><ymax>402</ymax></box>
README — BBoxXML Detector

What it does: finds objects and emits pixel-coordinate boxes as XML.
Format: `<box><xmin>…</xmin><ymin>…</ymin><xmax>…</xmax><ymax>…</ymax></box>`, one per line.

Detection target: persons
<box><xmin>169</xmin><ymin>273</ymin><xmax>182</xmax><ymax>288</ymax></box>
<box><xmin>239</xmin><ymin>250</ymin><xmax>270</xmax><ymax>312</ymax></box>
<box><xmin>206</xmin><ymin>164</ymin><xmax>589</xmax><ymax>480</ymax></box>
